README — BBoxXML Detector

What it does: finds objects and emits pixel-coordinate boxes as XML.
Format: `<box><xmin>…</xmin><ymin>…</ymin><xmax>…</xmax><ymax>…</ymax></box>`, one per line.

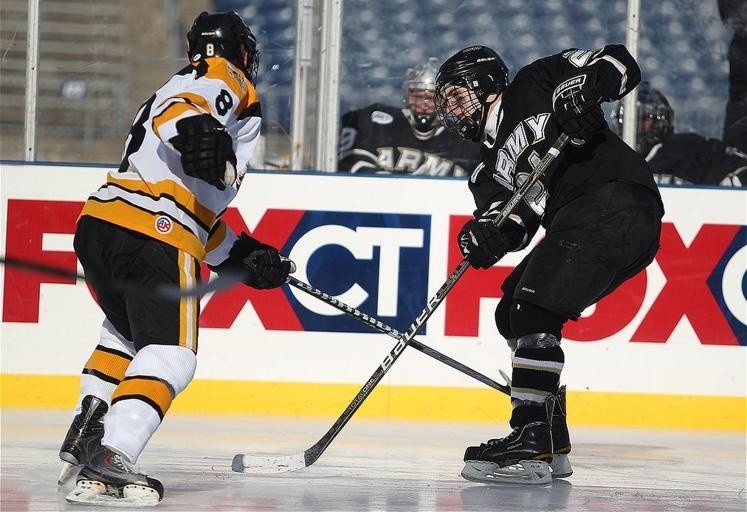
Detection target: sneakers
<box><xmin>462</xmin><ymin>383</ymin><xmax>574</xmax><ymax>467</ymax></box>
<box><xmin>56</xmin><ymin>394</ymin><xmax>111</xmax><ymax>469</ymax></box>
<box><xmin>72</xmin><ymin>443</ymin><xmax>165</xmax><ymax>501</ymax></box>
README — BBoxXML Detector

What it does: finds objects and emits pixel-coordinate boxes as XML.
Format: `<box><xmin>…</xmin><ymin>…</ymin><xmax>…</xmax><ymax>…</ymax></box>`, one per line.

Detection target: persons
<box><xmin>610</xmin><ymin>79</ymin><xmax>747</xmax><ymax>186</ymax></box>
<box><xmin>430</xmin><ymin>42</ymin><xmax>665</xmax><ymax>469</ymax></box>
<box><xmin>58</xmin><ymin>6</ymin><xmax>300</xmax><ymax>500</ymax></box>
<box><xmin>338</xmin><ymin>57</ymin><xmax>483</xmax><ymax>177</ymax></box>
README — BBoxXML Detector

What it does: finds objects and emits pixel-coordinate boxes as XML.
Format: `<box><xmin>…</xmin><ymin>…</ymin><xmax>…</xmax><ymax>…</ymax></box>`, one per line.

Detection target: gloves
<box><xmin>456</xmin><ymin>218</ymin><xmax>519</xmax><ymax>270</ymax></box>
<box><xmin>550</xmin><ymin>66</ymin><xmax>609</xmax><ymax>144</ymax></box>
<box><xmin>204</xmin><ymin>231</ymin><xmax>298</xmax><ymax>289</ymax></box>
<box><xmin>166</xmin><ymin>110</ymin><xmax>239</xmax><ymax>192</ymax></box>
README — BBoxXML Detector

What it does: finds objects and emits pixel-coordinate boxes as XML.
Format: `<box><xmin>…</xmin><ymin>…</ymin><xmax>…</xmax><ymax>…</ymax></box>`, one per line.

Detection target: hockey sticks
<box><xmin>285</xmin><ymin>276</ymin><xmax>512</xmax><ymax>396</ymax></box>
<box><xmin>233</xmin><ymin>131</ymin><xmax>569</xmax><ymax>472</ymax></box>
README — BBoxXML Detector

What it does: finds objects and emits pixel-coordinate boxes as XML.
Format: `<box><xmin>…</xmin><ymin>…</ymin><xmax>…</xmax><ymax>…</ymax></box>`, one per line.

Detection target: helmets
<box><xmin>400</xmin><ymin>55</ymin><xmax>448</xmax><ymax>125</ymax></box>
<box><xmin>610</xmin><ymin>78</ymin><xmax>676</xmax><ymax>147</ymax></box>
<box><xmin>433</xmin><ymin>45</ymin><xmax>510</xmax><ymax>128</ymax></box>
<box><xmin>185</xmin><ymin>9</ymin><xmax>260</xmax><ymax>88</ymax></box>
